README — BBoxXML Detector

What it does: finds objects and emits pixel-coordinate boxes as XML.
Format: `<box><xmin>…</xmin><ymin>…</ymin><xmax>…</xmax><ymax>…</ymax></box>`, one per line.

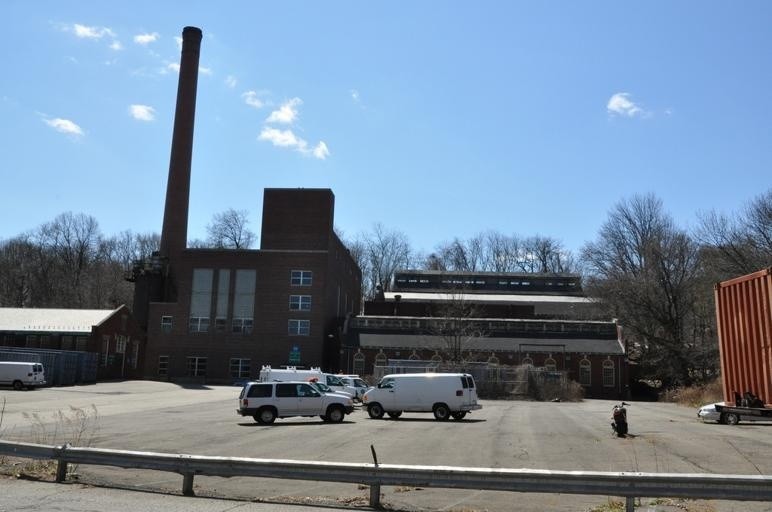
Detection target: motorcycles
<box><xmin>610</xmin><ymin>402</ymin><xmax>631</xmax><ymax>437</ymax></box>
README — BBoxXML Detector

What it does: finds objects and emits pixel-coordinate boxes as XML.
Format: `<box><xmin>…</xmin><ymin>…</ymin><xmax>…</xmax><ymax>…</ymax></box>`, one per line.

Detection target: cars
<box><xmin>696</xmin><ymin>390</ymin><xmax>771</xmax><ymax>425</ymax></box>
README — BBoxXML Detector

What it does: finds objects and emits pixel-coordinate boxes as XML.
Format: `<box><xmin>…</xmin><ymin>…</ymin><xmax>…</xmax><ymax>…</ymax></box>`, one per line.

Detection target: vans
<box><xmin>236</xmin><ymin>379</ymin><xmax>354</xmax><ymax>425</ymax></box>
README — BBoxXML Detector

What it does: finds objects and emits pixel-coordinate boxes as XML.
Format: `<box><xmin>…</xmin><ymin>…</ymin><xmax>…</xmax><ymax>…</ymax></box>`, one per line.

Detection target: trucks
<box><xmin>360</xmin><ymin>372</ymin><xmax>482</xmax><ymax>421</ymax></box>
<box><xmin>1</xmin><ymin>360</ymin><xmax>47</xmax><ymax>390</ymax></box>
<box><xmin>260</xmin><ymin>365</ymin><xmax>375</xmax><ymax>404</ymax></box>
